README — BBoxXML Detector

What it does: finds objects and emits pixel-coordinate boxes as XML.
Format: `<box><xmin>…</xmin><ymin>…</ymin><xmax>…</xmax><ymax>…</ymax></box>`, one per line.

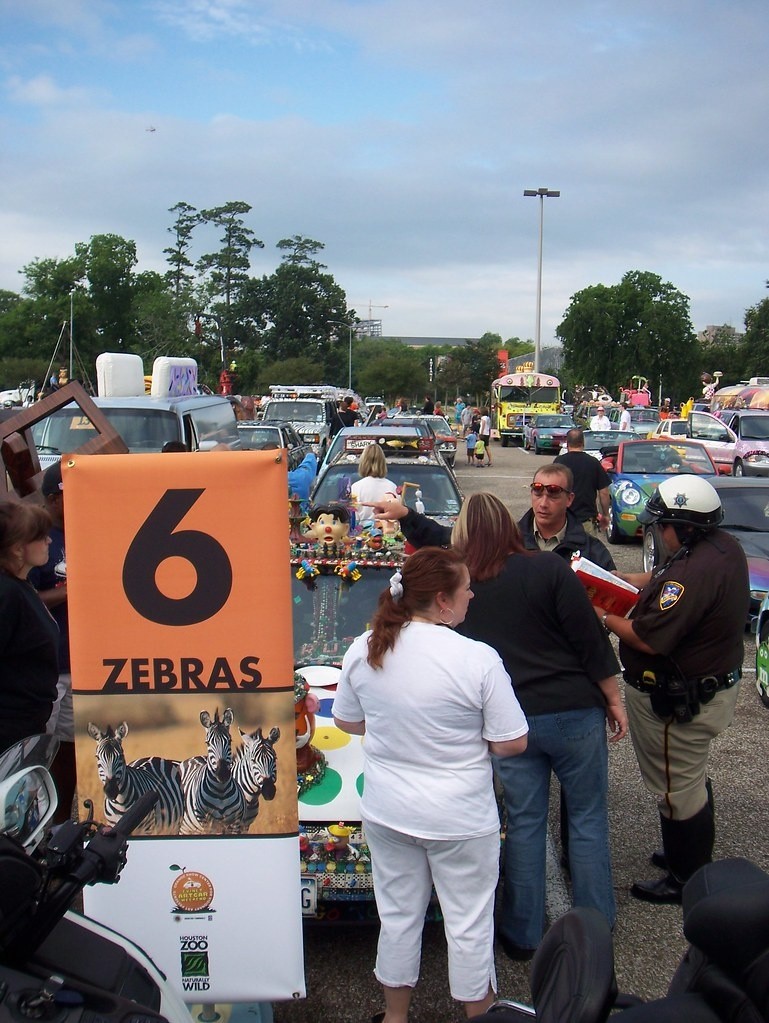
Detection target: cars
<box><xmin>640</xmin><ymin>477</ymin><xmax>768</xmax><ymax>622</ymax></box>
<box><xmin>691</xmin><ymin>402</ymin><xmax>710</xmax><ymax>425</ymax></box>
<box><xmin>608</xmin><ymin>408</ymin><xmax>661</xmax><ymax>440</ymax></box>
<box><xmin>310</xmin><ymin>413</ymin><xmax>465</xmax><ymax>533</ymax></box>
<box><xmin>291</xmin><ymin>541</ymin><xmax>444</xmax><ymax>926</ymax></box>
<box><xmin>689</xmin><ymin>405</ymin><xmax>769</xmax><ymax>478</ymax></box>
<box><xmin>558</xmin><ymin>430</ymin><xmax>644</xmax><ymax>461</ymax></box>
<box><xmin>237</xmin><ymin>420</ymin><xmax>312</xmax><ymax>468</ymax></box>
<box><xmin>564</xmin><ymin>405</ymin><xmax>616</xmax><ymax>430</ymax></box>
<box><xmin>523</xmin><ymin>414</ymin><xmax>582</xmax><ymax>455</ymax></box>
<box><xmin>364</xmin><ymin>397</ymin><xmax>385</xmax><ymax>410</ymax></box>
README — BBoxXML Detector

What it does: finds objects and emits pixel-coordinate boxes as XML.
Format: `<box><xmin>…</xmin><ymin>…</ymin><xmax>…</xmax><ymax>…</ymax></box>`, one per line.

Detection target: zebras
<box><xmin>86</xmin><ymin>706</ymin><xmax>280</xmax><ymax>836</ymax></box>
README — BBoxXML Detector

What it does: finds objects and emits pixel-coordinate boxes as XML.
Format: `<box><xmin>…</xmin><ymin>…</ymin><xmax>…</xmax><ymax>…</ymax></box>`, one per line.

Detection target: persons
<box><xmin>617</xmin><ymin>402</ymin><xmax>631</xmax><ymax>439</ymax></box>
<box><xmin>378</xmin><ymin>394</ymin><xmax>494</xmax><ymax>468</ymax></box>
<box><xmin>50</xmin><ymin>372</ymin><xmax>57</xmax><ymax>387</ymax></box>
<box><xmin>451</xmin><ymin>492</ymin><xmax>628</xmax><ymax>962</ymax></box>
<box><xmin>22</xmin><ymin>396</ymin><xmax>33</xmax><ymax>407</ymax></box>
<box><xmin>288</xmin><ymin>447</ymin><xmax>318</xmax><ymax>512</ymax></box>
<box><xmin>361</xmin><ymin>464</ymin><xmax>617</xmax><ymax>572</ymax></box>
<box><xmin>661</xmin><ymin>398</ymin><xmax>685</xmax><ymax>419</ymax></box>
<box><xmin>701</xmin><ymin>372</ymin><xmax>723</xmax><ymax>398</ymax></box>
<box><xmin>0</xmin><ymin>461</ymin><xmax>75</xmax><ymax>778</ymax></box>
<box><xmin>332</xmin><ymin>547</ymin><xmax>529</xmax><ymax>1023</ymax></box>
<box><xmin>592</xmin><ymin>474</ymin><xmax>749</xmax><ymax>904</ymax></box>
<box><xmin>351</xmin><ymin>444</ymin><xmax>403</xmax><ymax>520</ymax></box>
<box><xmin>328</xmin><ymin>396</ymin><xmax>359</xmax><ymax>449</ymax></box>
<box><xmin>307</xmin><ymin>503</ymin><xmax>350</xmax><ymax>548</ymax></box>
<box><xmin>590</xmin><ymin>407</ymin><xmax>611</xmax><ymax>439</ymax></box>
<box><xmin>553</xmin><ymin>429</ymin><xmax>613</xmax><ymax>537</ymax></box>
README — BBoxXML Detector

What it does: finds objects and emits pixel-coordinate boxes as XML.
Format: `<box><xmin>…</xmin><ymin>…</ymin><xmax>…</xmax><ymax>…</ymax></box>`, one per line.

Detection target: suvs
<box><xmin>258</xmin><ymin>398</ymin><xmax>336</xmax><ymax>450</ymax></box>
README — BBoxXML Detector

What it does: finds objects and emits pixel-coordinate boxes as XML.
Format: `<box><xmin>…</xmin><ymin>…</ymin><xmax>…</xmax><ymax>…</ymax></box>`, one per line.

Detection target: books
<box><xmin>571</xmin><ymin>557</ymin><xmax>640</xmax><ymax>619</ymax></box>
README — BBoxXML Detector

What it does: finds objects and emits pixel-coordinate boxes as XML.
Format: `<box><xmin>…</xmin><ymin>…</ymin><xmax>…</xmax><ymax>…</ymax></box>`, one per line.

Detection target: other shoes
<box><xmin>497</xmin><ymin>922</ymin><xmax>538</xmax><ymax>960</ymax></box>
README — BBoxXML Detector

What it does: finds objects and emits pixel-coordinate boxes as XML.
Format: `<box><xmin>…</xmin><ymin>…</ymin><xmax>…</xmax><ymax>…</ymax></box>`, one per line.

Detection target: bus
<box><xmin>490</xmin><ymin>373</ymin><xmax>561</xmax><ymax>444</ymax></box>
<box><xmin>710</xmin><ymin>377</ymin><xmax>769</xmax><ymax>412</ymax></box>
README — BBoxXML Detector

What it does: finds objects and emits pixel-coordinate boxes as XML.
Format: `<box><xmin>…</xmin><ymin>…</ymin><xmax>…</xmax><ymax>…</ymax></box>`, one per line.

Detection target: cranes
<box><xmin>347</xmin><ymin>300</ymin><xmax>388</xmax><ymax>319</ymax></box>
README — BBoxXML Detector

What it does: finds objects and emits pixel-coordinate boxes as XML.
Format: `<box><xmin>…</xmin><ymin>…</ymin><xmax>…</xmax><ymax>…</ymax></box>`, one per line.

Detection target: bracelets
<box><xmin>601</xmin><ymin>612</ymin><xmax>613</xmax><ymax>633</ymax></box>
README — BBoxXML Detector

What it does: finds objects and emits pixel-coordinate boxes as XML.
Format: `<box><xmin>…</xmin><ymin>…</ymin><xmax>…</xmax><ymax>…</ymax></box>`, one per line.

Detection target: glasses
<box><xmin>529</xmin><ymin>482</ymin><xmax>572</xmax><ymax>496</ymax></box>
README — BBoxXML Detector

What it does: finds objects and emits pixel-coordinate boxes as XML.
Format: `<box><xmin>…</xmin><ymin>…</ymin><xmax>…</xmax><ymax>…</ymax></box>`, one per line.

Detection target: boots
<box><xmin>631</xmin><ymin>778</ymin><xmax>715</xmax><ymax>904</ymax></box>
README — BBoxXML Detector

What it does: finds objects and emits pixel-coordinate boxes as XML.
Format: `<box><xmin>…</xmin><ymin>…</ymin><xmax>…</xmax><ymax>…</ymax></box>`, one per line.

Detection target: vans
<box><xmin>26</xmin><ymin>354</ymin><xmax>243</xmax><ymax>470</ymax></box>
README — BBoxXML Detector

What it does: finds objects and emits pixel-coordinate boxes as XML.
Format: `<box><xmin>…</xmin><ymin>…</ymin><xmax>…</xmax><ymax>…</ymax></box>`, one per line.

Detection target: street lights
<box><xmin>524</xmin><ymin>189</ymin><xmax>561</xmax><ymax>371</ymax></box>
<box><xmin>327</xmin><ymin>320</ymin><xmax>380</xmax><ymax>390</ymax></box>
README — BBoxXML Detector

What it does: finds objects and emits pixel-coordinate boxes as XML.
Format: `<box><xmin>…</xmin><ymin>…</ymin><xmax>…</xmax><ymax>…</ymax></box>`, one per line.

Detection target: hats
<box><xmin>42</xmin><ymin>462</ymin><xmax>64</xmax><ymax>496</ymax></box>
<box><xmin>597</xmin><ymin>407</ymin><xmax>604</xmax><ymax>411</ymax></box>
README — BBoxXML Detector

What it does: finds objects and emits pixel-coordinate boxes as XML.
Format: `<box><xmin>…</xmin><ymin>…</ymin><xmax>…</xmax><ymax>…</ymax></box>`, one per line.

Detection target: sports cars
<box><xmin>599</xmin><ymin>440</ymin><xmax>731</xmax><ymax>543</ymax></box>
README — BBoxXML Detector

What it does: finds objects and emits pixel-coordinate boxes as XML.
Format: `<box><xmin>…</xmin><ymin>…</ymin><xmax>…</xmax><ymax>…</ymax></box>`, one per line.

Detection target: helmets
<box><xmin>635</xmin><ymin>474</ymin><xmax>724</xmax><ymax>527</ymax></box>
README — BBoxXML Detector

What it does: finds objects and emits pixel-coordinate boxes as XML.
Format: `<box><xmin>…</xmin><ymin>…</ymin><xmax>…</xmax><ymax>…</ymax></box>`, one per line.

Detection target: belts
<box><xmin>621</xmin><ymin>669</ymin><xmax>741</xmax><ymax>694</ymax></box>
